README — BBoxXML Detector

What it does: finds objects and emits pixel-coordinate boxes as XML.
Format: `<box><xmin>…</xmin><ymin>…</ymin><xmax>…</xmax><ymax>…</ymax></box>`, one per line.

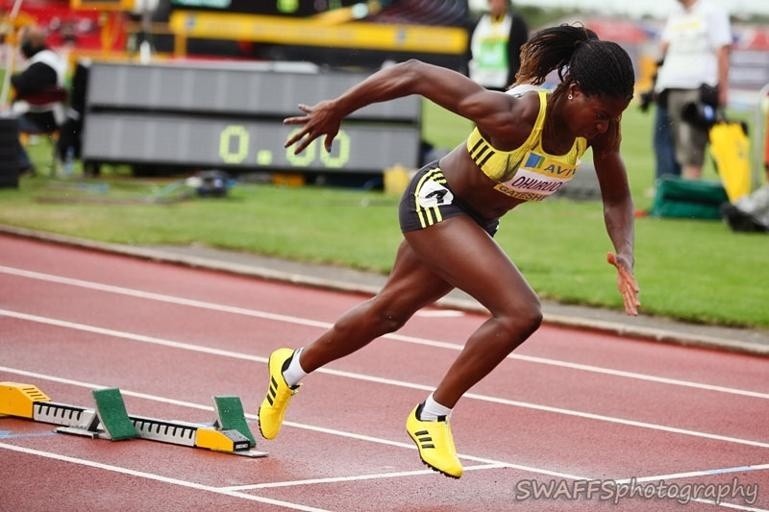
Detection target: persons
<box><xmin>258</xmin><ymin>26</ymin><xmax>641</xmax><ymax>479</ymax></box>
<box><xmin>467</xmin><ymin>0</ymin><xmax>528</xmax><ymax>91</ymax></box>
<box><xmin>0</xmin><ymin>27</ymin><xmax>68</xmax><ymax>188</ymax></box>
<box><xmin>640</xmin><ymin>0</ymin><xmax>733</xmax><ymax>183</ymax></box>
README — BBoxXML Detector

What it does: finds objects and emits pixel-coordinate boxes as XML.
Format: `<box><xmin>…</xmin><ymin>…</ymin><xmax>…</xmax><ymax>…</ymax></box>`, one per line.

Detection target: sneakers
<box><xmin>258</xmin><ymin>348</ymin><xmax>303</xmax><ymax>440</ymax></box>
<box><xmin>405</xmin><ymin>403</ymin><xmax>463</xmax><ymax>479</ymax></box>
